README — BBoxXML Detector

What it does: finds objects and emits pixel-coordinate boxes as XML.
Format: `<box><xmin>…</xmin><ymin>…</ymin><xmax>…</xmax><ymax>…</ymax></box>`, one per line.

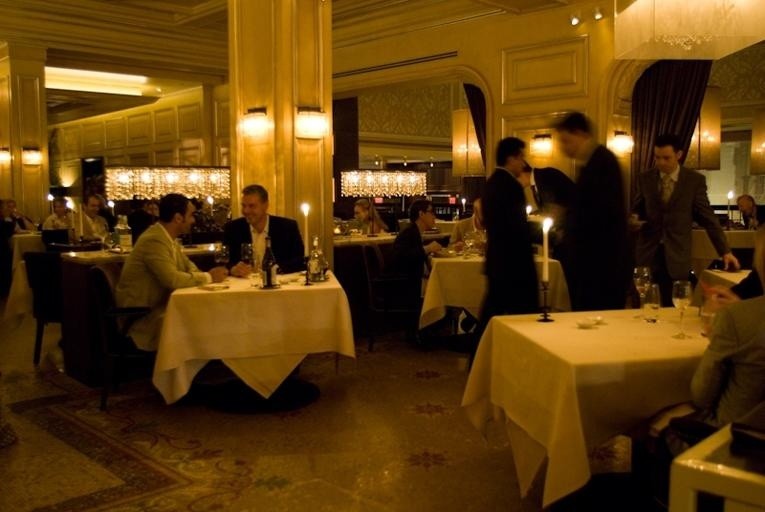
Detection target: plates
<box><xmin>575</xmin><ymin>315</ymin><xmax>606</xmax><ymax>329</ymax></box>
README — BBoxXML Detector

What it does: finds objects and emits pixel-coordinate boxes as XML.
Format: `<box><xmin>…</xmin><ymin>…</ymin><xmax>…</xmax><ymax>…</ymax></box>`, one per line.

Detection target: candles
<box><xmin>541</xmin><ymin>217</ymin><xmax>551</xmax><ymax>283</ymax></box>
<box><xmin>727</xmin><ymin>191</ymin><xmax>734</xmax><ymax>218</ymax></box>
<box><xmin>303</xmin><ymin>212</ymin><xmax>310</xmax><ymax>257</ymax></box>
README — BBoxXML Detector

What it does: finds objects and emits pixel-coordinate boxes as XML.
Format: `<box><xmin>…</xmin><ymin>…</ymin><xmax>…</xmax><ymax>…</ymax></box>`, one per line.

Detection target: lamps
<box><xmin>615</xmin><ymin>130</ymin><xmax>635</xmax><ymax>155</ymax></box>
<box><xmin>750</xmin><ymin>107</ymin><xmax>765</xmax><ymax>177</ymax></box>
<box><xmin>612</xmin><ymin>1</ymin><xmax>765</xmax><ymax>62</ymax></box>
<box><xmin>295</xmin><ymin>106</ymin><xmax>327</xmax><ymax>142</ymax></box>
<box><xmin>339</xmin><ymin>167</ymin><xmax>428</xmax><ymax>200</ymax></box>
<box><xmin>683</xmin><ymin>84</ymin><xmax>722</xmax><ymax>171</ymax></box>
<box><xmin>238</xmin><ymin>106</ymin><xmax>272</xmax><ymax>143</ymax></box>
<box><xmin>101</xmin><ymin>162</ymin><xmax>231</xmax><ymax>203</ymax></box>
<box><xmin>529</xmin><ymin>133</ymin><xmax>553</xmax><ymax>156</ymax></box>
<box><xmin>450</xmin><ymin>107</ymin><xmax>485</xmax><ymax>178</ymax></box>
<box><xmin>21</xmin><ymin>145</ymin><xmax>42</xmax><ymax>166</ymax></box>
<box><xmin>0</xmin><ymin>147</ymin><xmax>10</xmax><ymax>167</ymax></box>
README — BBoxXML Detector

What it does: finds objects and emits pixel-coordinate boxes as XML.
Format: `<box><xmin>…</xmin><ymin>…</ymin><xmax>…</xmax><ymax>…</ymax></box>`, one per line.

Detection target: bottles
<box><xmin>308</xmin><ymin>233</ymin><xmax>328</xmax><ymax>282</ymax></box>
<box><xmin>261</xmin><ymin>237</ymin><xmax>278</xmax><ymax>289</ymax></box>
<box><xmin>113</xmin><ymin>214</ymin><xmax>133</xmax><ymax>254</ymax></box>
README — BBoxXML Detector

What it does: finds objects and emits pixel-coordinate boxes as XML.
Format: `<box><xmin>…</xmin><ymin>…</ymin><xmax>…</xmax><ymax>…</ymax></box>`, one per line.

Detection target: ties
<box><xmin>255</xmin><ymin>233</ymin><xmax>264</xmax><ymax>266</ymax></box>
<box><xmin>661</xmin><ymin>177</ymin><xmax>673</xmax><ymax>203</ymax></box>
<box><xmin>530</xmin><ymin>184</ymin><xmax>540</xmax><ymax>208</ymax></box>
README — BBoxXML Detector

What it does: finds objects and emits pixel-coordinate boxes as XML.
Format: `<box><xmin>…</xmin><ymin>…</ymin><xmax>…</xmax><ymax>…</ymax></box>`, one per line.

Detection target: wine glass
<box><xmin>239</xmin><ymin>242</ymin><xmax>255</xmax><ymax>279</ymax></box>
<box><xmin>213</xmin><ymin>244</ymin><xmax>230</xmax><ymax>282</ymax></box>
<box><xmin>633</xmin><ymin>264</ymin><xmax>652</xmax><ymax>319</ymax></box>
<box><xmin>670</xmin><ymin>279</ymin><xmax>693</xmax><ymax>340</ymax></box>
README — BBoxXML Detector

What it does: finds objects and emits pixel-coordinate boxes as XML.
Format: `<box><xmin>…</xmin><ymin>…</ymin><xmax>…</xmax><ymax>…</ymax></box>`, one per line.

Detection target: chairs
<box><xmin>4</xmin><ymin>220</ymin><xmax>111</xmax><ymax>317</ymax></box>
<box><xmin>22</xmin><ymin>250</ymin><xmax>71</xmax><ymax>365</ymax></box>
<box><xmin>90</xmin><ymin>267</ymin><xmax>163</xmax><ymax>407</ymax></box>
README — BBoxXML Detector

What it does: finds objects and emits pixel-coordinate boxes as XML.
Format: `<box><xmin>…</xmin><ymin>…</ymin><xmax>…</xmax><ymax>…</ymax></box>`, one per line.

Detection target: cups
<box><xmin>643</xmin><ymin>284</ymin><xmax>661</xmax><ymax>324</ymax></box>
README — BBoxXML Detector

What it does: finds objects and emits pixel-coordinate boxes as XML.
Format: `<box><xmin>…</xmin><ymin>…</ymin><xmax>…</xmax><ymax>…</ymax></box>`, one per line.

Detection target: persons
<box><xmin>0</xmin><ymin>193</ymin><xmax>230</xmax><ymax>312</ymax></box>
<box><xmin>629</xmin><ymin>225</ymin><xmax>765</xmax><ymax>512</ymax></box>
<box><xmin>466</xmin><ymin>138</ymin><xmax>543</xmax><ymax>372</ymax></box>
<box><xmin>627</xmin><ymin>132</ymin><xmax>743</xmax><ymax>307</ymax></box>
<box><xmin>375</xmin><ymin>199</ymin><xmax>453</xmax><ymax>350</ymax></box>
<box><xmin>351</xmin><ymin>200</ymin><xmax>390</xmax><ymax>235</ymax></box>
<box><xmin>215</xmin><ymin>183</ymin><xmax>305</xmax><ymax>277</ymax></box>
<box><xmin>115</xmin><ymin>194</ymin><xmax>230</xmax><ymax>399</ymax></box>
<box><xmin>448</xmin><ymin>198</ymin><xmax>485</xmax><ymax>250</ymax></box>
<box><xmin>548</xmin><ymin>109</ymin><xmax>641</xmax><ymax>311</ymax></box>
<box><xmin>735</xmin><ymin>194</ymin><xmax>765</xmax><ymax>228</ymax></box>
<box><xmin>512</xmin><ymin>160</ymin><xmax>577</xmax><ymax>216</ymax></box>
